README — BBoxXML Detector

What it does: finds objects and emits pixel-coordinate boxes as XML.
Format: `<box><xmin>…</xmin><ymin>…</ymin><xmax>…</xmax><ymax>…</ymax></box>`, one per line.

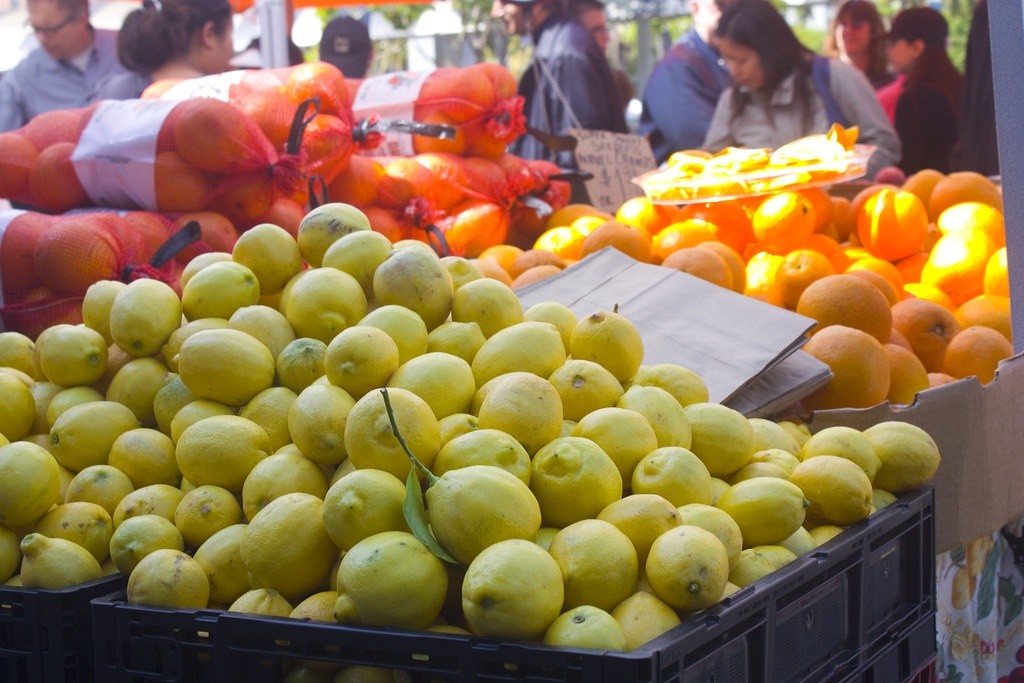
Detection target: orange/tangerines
<box><xmin>0</xmin><ymin>61</ymin><xmax>1013</xmax><ymax>413</ymax></box>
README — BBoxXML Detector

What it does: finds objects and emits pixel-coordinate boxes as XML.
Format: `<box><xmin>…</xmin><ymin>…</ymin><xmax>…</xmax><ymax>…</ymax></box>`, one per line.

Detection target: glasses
<box><xmin>23</xmin><ymin>16</ymin><xmax>75</xmax><ymax>38</ymax></box>
<box><xmin>838</xmin><ymin>17</ymin><xmax>870</xmax><ymax>32</ymax></box>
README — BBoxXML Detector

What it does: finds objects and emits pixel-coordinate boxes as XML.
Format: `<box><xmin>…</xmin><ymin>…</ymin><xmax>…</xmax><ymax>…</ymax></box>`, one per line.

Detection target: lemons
<box><xmin>0</xmin><ymin>204</ymin><xmax>939</xmax><ymax>683</ymax></box>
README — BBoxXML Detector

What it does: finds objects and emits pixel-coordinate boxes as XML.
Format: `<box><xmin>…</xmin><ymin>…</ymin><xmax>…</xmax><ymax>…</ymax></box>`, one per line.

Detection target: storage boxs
<box><xmin>785</xmin><ymin>348</ymin><xmax>1023</xmax><ymax>682</ymax></box>
<box><xmin>0</xmin><ymin>481</ymin><xmax>937</xmax><ymax>683</ymax></box>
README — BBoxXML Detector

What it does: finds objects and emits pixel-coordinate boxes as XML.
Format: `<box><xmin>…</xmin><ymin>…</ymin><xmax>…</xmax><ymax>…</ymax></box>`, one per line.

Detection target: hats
<box><xmin>881</xmin><ymin>8</ymin><xmax>948</xmax><ymax>45</ymax></box>
<box><xmin>319</xmin><ymin>16</ymin><xmax>370</xmax><ymax>78</ymax></box>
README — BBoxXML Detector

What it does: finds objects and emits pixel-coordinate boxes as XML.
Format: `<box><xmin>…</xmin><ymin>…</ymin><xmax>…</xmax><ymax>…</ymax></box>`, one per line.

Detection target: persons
<box><xmin>114</xmin><ymin>1</ymin><xmax>239</xmax><ymax>97</ymax></box>
<box><xmin>0</xmin><ymin>0</ymin><xmax>151</xmax><ymax>134</ymax></box>
<box><xmin>487</xmin><ymin>0</ymin><xmax>999</xmax><ymax>212</ymax></box>
